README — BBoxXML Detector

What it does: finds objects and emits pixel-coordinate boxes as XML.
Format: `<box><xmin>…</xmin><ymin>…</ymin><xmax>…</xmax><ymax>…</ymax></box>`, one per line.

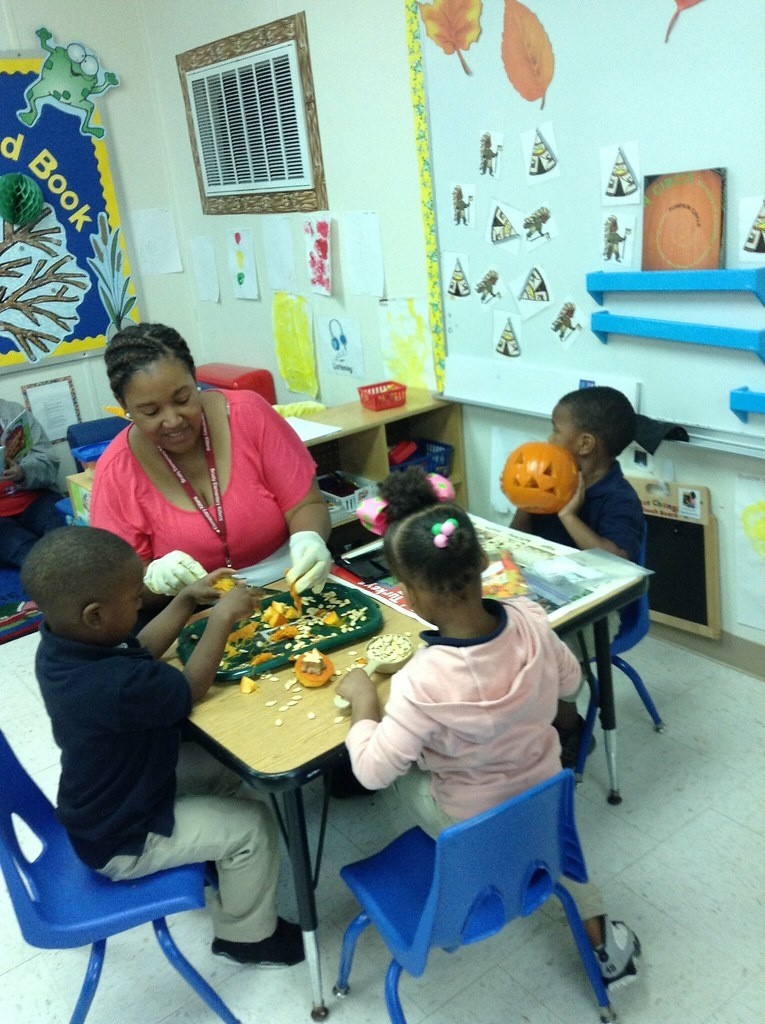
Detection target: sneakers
<box><xmin>212</xmin><ymin>917</ymin><xmax>306</xmax><ymax>968</ymax></box>
<box><xmin>593</xmin><ymin>915</ymin><xmax>642</xmax><ymax>993</ymax></box>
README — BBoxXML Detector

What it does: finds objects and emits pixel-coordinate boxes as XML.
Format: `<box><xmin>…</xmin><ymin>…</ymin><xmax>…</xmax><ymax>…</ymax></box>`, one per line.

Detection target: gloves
<box><xmin>284</xmin><ymin>531</ymin><xmax>332</xmax><ymax>594</ymax></box>
<box><xmin>142</xmin><ymin>550</ymin><xmax>207</xmax><ymax>597</ymax></box>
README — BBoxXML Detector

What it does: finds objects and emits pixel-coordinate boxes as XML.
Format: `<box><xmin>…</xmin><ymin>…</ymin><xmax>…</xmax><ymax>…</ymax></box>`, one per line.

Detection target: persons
<box><xmin>0</xmin><ymin>399</ymin><xmax>68</xmax><ymax>566</ymax></box>
<box><xmin>336</xmin><ymin>463</ymin><xmax>641</xmax><ymax>990</ymax></box>
<box><xmin>500</xmin><ymin>386</ymin><xmax>644</xmax><ymax>770</ymax></box>
<box><xmin>89</xmin><ymin>321</ymin><xmax>332</xmax><ymax>596</ymax></box>
<box><xmin>21</xmin><ymin>526</ymin><xmax>305</xmax><ymax>968</ymax></box>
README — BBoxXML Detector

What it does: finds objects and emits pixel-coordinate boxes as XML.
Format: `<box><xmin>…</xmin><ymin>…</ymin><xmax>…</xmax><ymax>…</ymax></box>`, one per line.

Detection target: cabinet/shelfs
<box><xmin>62</xmin><ymin>389</ymin><xmax>468</xmax><ymax>560</ymax></box>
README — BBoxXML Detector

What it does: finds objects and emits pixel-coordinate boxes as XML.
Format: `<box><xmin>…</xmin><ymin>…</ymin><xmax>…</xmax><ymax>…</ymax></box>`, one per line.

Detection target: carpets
<box><xmin>0</xmin><ymin>544</ymin><xmax>45</xmax><ymax>648</ymax></box>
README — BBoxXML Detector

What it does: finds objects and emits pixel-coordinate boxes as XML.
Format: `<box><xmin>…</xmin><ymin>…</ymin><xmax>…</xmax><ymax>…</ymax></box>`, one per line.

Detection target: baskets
<box><xmin>390</xmin><ymin>439</ymin><xmax>454</xmax><ymax>481</ymax></box>
<box><xmin>315</xmin><ymin>471</ymin><xmax>378</xmax><ymax>515</ymax></box>
<box><xmin>357</xmin><ymin>380</ymin><xmax>407</xmax><ymax>410</ymax></box>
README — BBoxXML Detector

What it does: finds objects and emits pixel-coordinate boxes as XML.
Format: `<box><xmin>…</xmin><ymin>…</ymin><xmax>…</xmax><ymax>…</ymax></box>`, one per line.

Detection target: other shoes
<box><xmin>560</xmin><ymin>731</ymin><xmax>596</xmax><ymax>766</ymax></box>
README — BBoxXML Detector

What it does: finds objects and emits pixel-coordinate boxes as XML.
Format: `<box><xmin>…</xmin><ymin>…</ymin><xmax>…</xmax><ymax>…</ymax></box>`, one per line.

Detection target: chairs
<box><xmin>0</xmin><ymin>725</ymin><xmax>248</xmax><ymax>1023</ymax></box>
<box><xmin>555</xmin><ymin>517</ymin><xmax>667</xmax><ymax>787</ymax></box>
<box><xmin>332</xmin><ymin>772</ymin><xmax>619</xmax><ymax>1022</ymax></box>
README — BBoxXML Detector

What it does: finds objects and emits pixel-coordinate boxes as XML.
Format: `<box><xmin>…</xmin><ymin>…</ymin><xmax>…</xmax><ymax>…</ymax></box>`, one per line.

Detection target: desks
<box><xmin>153</xmin><ymin>502</ymin><xmax>654</xmax><ymax>1024</ymax></box>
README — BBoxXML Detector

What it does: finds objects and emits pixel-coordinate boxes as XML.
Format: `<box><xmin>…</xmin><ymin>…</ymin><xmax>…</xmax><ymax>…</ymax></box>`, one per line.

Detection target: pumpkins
<box><xmin>295</xmin><ymin>651</ymin><xmax>335</xmax><ymax>687</ymax></box>
<box><xmin>262</xmin><ymin>602</ymin><xmax>340</xmax><ymax>637</ymax></box>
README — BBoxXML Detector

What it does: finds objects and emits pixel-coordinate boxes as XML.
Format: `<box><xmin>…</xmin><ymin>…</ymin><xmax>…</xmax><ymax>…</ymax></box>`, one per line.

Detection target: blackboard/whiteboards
<box><xmin>622</xmin><ymin>476</ymin><xmax>721</xmax><ymax>640</ymax></box>
<box><xmin>406</xmin><ymin>0</ymin><xmax>765</xmax><ymax>461</ymax></box>
